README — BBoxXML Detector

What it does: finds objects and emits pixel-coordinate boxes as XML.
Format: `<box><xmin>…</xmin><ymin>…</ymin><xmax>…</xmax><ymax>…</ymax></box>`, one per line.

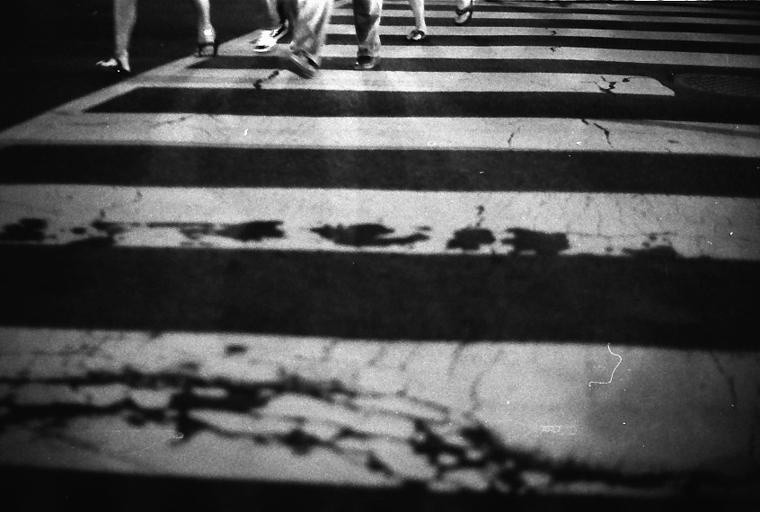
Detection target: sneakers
<box><xmin>254</xmin><ymin>19</ymin><xmax>290</xmax><ymax>52</ymax></box>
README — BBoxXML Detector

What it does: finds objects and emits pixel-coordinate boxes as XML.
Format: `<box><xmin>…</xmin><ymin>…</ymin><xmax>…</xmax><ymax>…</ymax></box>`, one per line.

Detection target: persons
<box><xmin>94</xmin><ymin>0</ymin><xmax>220</xmax><ymax>75</ymax></box>
<box><xmin>250</xmin><ymin>0</ymin><xmax>290</xmax><ymax>54</ymax></box>
<box><xmin>263</xmin><ymin>0</ymin><xmax>383</xmax><ymax>80</ymax></box>
<box><xmin>406</xmin><ymin>0</ymin><xmax>474</xmax><ymax>42</ymax></box>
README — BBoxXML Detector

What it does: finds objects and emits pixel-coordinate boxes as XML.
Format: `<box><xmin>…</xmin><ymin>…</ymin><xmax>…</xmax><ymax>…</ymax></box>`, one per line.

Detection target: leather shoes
<box><xmin>284</xmin><ymin>49</ymin><xmax>318</xmax><ymax>78</ymax></box>
<box><xmin>354</xmin><ymin>54</ymin><xmax>381</xmax><ymax>70</ymax></box>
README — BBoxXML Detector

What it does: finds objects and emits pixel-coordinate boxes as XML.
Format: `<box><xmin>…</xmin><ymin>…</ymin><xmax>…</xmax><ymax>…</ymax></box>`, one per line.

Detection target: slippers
<box><xmin>193</xmin><ymin>41</ymin><xmax>218</xmax><ymax>58</ymax></box>
<box><xmin>454</xmin><ymin>1</ymin><xmax>474</xmax><ymax>26</ymax></box>
<box><xmin>97</xmin><ymin>57</ymin><xmax>131</xmax><ymax>77</ymax></box>
<box><xmin>405</xmin><ymin>31</ymin><xmax>427</xmax><ymax>43</ymax></box>
